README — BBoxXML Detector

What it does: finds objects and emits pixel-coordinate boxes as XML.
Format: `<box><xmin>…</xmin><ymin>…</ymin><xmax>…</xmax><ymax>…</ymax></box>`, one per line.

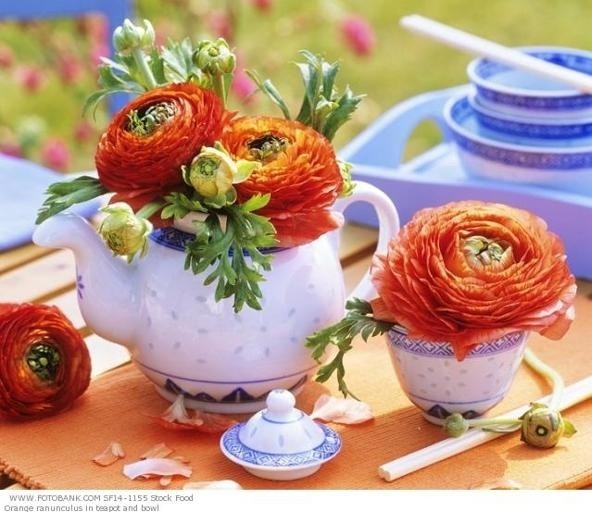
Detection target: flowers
<box><xmin>31</xmin><ymin>16</ymin><xmax>369</xmax><ymax>314</ymax></box>
<box><xmin>304</xmin><ymin>199</ymin><xmax>579</xmax><ymax>405</ymax></box>
<box><xmin>0</xmin><ymin>300</ymin><xmax>93</xmax><ymax>425</ymax></box>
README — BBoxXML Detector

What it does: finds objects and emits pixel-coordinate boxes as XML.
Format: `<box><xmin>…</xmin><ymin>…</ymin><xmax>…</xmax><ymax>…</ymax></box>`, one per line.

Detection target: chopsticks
<box><xmin>369</xmin><ymin>377</ymin><xmax>591</xmax><ymax>483</ymax></box>
<box><xmin>393</xmin><ymin>9</ymin><xmax>592</xmax><ymax>98</ymax></box>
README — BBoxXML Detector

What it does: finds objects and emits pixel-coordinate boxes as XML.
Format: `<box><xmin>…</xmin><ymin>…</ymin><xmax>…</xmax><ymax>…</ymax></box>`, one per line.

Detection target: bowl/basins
<box><xmin>381</xmin><ymin>320</ymin><xmax>530</xmax><ymax>427</ymax></box>
<box><xmin>441</xmin><ymin>44</ymin><xmax>591</xmax><ymax>188</ymax></box>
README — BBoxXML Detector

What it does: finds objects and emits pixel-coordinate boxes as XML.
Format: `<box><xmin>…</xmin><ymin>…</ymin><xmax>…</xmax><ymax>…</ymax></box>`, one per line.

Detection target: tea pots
<box><xmin>29</xmin><ymin>181</ymin><xmax>402</xmax><ymax>419</ymax></box>
